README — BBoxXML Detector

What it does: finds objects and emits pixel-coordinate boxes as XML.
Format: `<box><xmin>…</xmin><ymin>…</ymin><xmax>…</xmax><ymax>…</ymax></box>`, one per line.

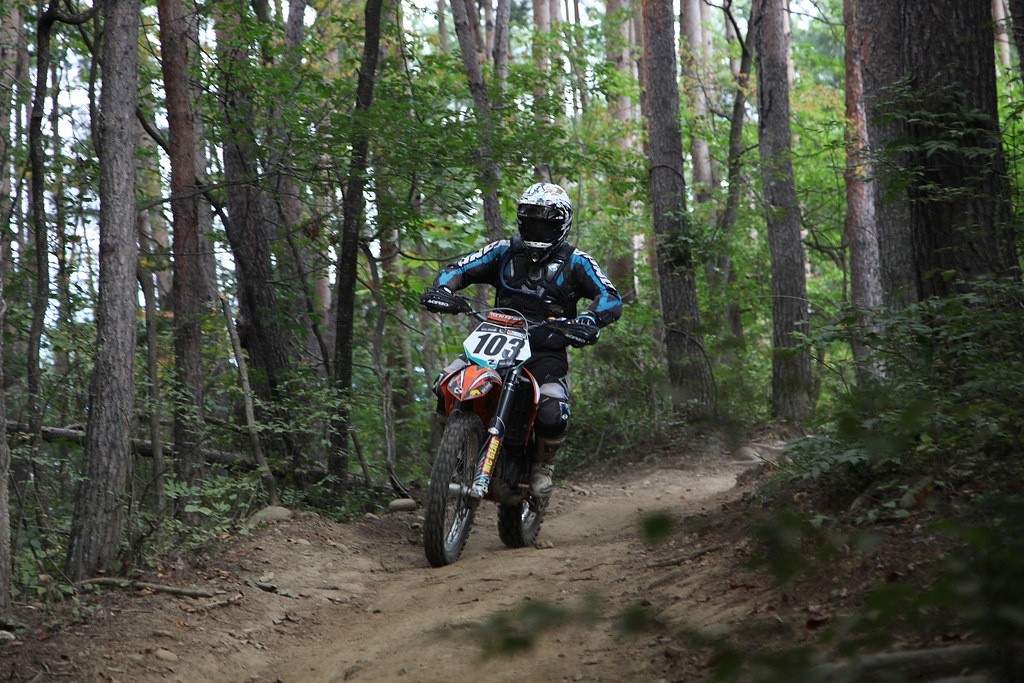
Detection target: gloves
<box><xmin>569</xmin><ymin>309</ymin><xmax>601</xmax><ymax>348</ymax></box>
<box><xmin>423</xmin><ymin>287</ymin><xmax>451</xmax><ymax>314</ymax></box>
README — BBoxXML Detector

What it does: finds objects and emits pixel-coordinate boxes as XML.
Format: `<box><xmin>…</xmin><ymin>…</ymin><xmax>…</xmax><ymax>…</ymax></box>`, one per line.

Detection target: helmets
<box><xmin>517</xmin><ymin>182</ymin><xmax>573</xmax><ymax>263</ymax></box>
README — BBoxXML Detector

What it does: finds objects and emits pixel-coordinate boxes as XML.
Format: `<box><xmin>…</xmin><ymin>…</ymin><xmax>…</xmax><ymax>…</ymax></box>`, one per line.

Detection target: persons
<box><xmin>426</xmin><ymin>183</ymin><xmax>622</xmax><ymax>498</ymax></box>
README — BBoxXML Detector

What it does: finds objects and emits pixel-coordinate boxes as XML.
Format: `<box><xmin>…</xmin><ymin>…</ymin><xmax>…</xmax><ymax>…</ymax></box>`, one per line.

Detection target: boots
<box><xmin>527</xmin><ymin>433</ymin><xmax>566</xmax><ymax>497</ymax></box>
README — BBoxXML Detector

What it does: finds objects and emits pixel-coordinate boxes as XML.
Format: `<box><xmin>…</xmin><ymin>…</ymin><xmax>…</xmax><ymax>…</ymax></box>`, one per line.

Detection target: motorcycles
<box><xmin>419</xmin><ymin>288</ymin><xmax>602</xmax><ymax>570</ymax></box>
